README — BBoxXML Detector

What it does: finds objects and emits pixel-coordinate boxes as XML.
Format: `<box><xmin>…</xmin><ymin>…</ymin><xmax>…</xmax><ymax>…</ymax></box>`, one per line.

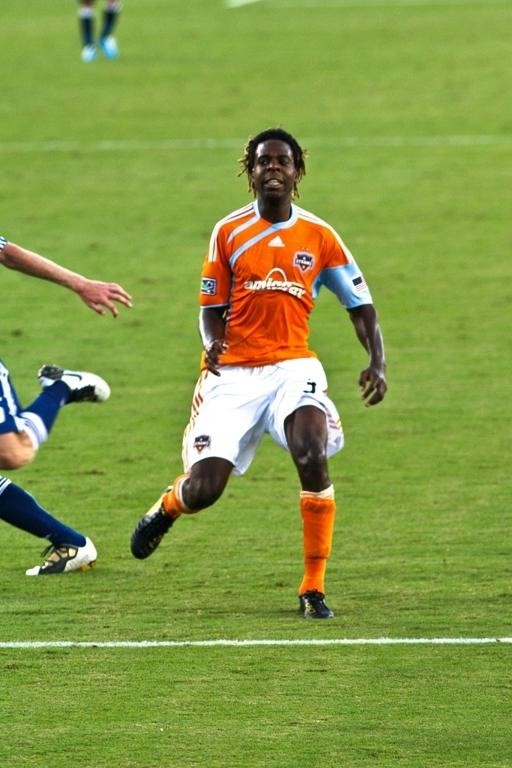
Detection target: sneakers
<box><xmin>26</xmin><ymin>537</ymin><xmax>97</xmax><ymax>575</ymax></box>
<box><xmin>299</xmin><ymin>590</ymin><xmax>332</xmax><ymax>619</ymax></box>
<box><xmin>131</xmin><ymin>486</ymin><xmax>182</xmax><ymax>559</ymax></box>
<box><xmin>38</xmin><ymin>365</ymin><xmax>111</xmax><ymax>403</ymax></box>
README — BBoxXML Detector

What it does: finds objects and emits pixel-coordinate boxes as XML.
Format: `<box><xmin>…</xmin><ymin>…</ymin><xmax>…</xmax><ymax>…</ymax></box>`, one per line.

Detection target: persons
<box><xmin>131</xmin><ymin>129</ymin><xmax>388</xmax><ymax>622</ymax></box>
<box><xmin>75</xmin><ymin>0</ymin><xmax>123</xmax><ymax>65</ymax></box>
<box><xmin>0</xmin><ymin>235</ymin><xmax>132</xmax><ymax>576</ymax></box>
